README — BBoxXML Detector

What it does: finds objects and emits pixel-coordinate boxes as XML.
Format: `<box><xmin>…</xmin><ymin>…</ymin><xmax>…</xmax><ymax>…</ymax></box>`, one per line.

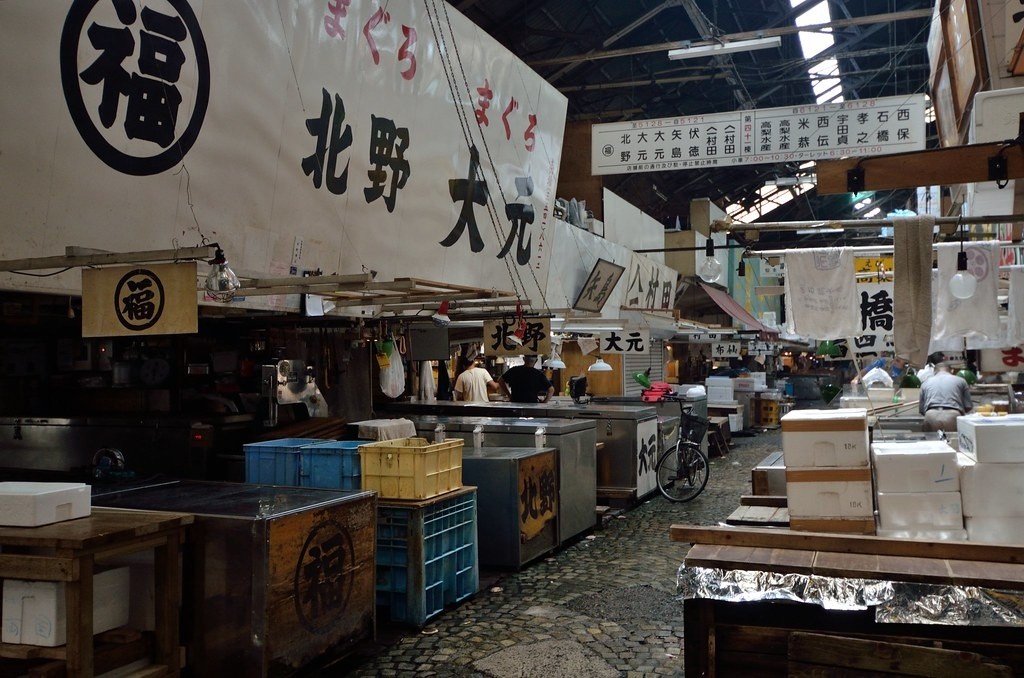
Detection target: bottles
<box><xmin>382</xmin><ymin>336</ymin><xmax>394</xmax><ymax>358</ymax></box>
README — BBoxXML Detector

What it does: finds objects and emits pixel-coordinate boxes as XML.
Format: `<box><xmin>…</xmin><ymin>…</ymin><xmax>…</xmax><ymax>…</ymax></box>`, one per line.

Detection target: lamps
<box><xmin>668</xmin><ymin>36</ymin><xmax>781</xmax><ymax>60</ymax></box>
<box><xmin>542</xmin><ymin>358</ymin><xmax>567</xmax><ymax>369</ymax></box>
<box><xmin>587</xmin><ymin>353</ymin><xmax>612</xmax><ymax>371</ymax></box>
<box><xmin>775</xmin><ymin>177</ymin><xmax>817</xmax><ymax>185</ymax></box>
<box><xmin>632</xmin><ymin>367</ymin><xmax>653</xmax><ymax>388</ymax></box>
<box><xmin>949</xmin><ymin>252</ymin><xmax>977</xmax><ymax>299</ymax></box>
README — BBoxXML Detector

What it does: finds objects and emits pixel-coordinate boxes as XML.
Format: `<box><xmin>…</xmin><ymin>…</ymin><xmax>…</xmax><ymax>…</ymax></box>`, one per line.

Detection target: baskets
<box><xmin>681</xmin><ymin>414</ymin><xmax>708</xmax><ymax>443</ymax></box>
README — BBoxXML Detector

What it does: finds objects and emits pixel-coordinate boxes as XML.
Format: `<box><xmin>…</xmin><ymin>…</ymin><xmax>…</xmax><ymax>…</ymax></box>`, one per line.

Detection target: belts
<box><xmin>929</xmin><ymin>406</ymin><xmax>964</xmax><ymax>416</ymax></box>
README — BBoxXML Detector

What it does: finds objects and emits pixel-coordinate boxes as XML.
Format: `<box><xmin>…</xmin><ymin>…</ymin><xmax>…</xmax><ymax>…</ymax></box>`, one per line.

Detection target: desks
<box><xmin>707</xmin><ymin>416</ymin><xmax>729</xmax><ymax>457</ymax></box>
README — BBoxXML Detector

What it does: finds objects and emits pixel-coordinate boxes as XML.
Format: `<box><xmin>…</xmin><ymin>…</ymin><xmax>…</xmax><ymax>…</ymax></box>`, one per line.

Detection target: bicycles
<box><xmin>656</xmin><ymin>392</ymin><xmax>712</xmax><ymax>502</ymax></box>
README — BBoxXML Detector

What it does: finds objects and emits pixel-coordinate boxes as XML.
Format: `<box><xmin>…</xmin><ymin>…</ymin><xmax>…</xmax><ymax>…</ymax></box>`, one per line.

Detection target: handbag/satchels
<box><xmin>379</xmin><ymin>332</ymin><xmax>406</xmax><ymax>397</ymax></box>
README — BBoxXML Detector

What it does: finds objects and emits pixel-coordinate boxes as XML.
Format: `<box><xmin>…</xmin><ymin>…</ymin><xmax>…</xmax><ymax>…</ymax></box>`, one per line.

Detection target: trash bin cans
<box><xmin>786</xmin><ymin>383</ymin><xmax>794</xmax><ymax>396</ymax></box>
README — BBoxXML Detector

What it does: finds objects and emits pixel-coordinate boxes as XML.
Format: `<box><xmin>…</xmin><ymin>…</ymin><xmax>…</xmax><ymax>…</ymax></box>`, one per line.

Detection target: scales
<box><xmin>140</xmin><ymin>346</ymin><xmax>177</xmax><ymax>389</ymax></box>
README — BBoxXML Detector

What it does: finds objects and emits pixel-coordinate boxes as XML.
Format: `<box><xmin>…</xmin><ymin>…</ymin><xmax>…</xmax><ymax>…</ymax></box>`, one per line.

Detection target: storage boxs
<box><xmin>780</xmin><ymin>409</ymin><xmax>1024</xmax><ymax>548</ymax></box>
<box><xmin>704</xmin><ymin>372</ymin><xmax>766</xmax><ymax>403</ymax></box>
<box><xmin>241</xmin><ymin>436</ymin><xmax>480</xmax><ymax>626</ymax></box>
<box><xmin>0</xmin><ymin>567</ymin><xmax>130</xmax><ymax>648</ymax></box>
<box><xmin>0</xmin><ymin>481</ymin><xmax>91</xmax><ymax>527</ymax></box>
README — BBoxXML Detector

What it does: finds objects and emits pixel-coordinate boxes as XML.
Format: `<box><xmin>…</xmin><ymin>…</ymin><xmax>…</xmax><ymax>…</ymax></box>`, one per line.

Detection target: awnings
<box><xmin>699</xmin><ymin>283</ymin><xmax>781</xmax><ymax>342</ymax></box>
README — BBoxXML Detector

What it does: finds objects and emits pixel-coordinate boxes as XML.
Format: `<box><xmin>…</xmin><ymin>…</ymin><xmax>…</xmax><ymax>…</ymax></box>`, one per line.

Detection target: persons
<box><xmin>497</xmin><ymin>356</ymin><xmax>554</xmax><ymax>403</ymax></box>
<box><xmin>919</xmin><ymin>363</ymin><xmax>973</xmax><ymax>433</ymax></box>
<box><xmin>454</xmin><ymin>356</ymin><xmax>499</xmax><ymax>402</ymax></box>
<box><xmin>851</xmin><ymin>355</ymin><xmax>913</xmax><ymax>384</ymax></box>
<box><xmin>916</xmin><ymin>351</ymin><xmax>945</xmax><ymax>383</ymax></box>
